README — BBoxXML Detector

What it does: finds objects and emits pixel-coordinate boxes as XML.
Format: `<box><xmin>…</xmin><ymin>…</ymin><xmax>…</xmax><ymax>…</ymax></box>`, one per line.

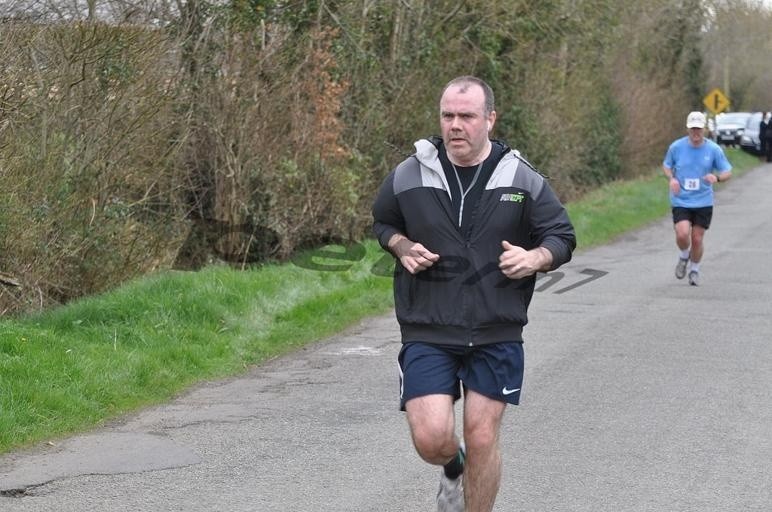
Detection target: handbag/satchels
<box><xmin>713</xmin><ymin>174</ymin><xmax>721</xmax><ymax>183</ymax></box>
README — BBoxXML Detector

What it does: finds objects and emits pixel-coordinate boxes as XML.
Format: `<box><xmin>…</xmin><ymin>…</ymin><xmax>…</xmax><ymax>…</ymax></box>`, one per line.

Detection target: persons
<box><xmin>758</xmin><ymin>112</ymin><xmax>768</xmax><ymax>161</ymax></box>
<box><xmin>661</xmin><ymin>109</ymin><xmax>733</xmax><ymax>285</ymax></box>
<box><xmin>371</xmin><ymin>76</ymin><xmax>576</xmax><ymax>512</ymax></box>
<box><xmin>764</xmin><ymin>117</ymin><xmax>772</xmax><ymax>164</ymax></box>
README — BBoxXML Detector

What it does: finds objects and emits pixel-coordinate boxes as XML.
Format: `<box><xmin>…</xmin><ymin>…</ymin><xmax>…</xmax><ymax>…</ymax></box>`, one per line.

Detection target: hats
<box><xmin>714</xmin><ymin>112</ymin><xmax>772</xmax><ymax>162</ymax></box>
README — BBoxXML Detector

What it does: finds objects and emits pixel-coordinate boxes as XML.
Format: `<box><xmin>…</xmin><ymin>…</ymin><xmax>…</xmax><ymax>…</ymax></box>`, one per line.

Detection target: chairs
<box><xmin>688</xmin><ymin>269</ymin><xmax>699</xmax><ymax>285</ymax></box>
<box><xmin>435</xmin><ymin>442</ymin><xmax>466</xmax><ymax>512</ymax></box>
<box><xmin>675</xmin><ymin>251</ymin><xmax>690</xmax><ymax>279</ymax></box>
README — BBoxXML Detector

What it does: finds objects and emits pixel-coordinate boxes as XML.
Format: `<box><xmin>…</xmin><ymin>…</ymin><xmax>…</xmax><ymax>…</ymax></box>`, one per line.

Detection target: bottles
<box><xmin>687</xmin><ymin>111</ymin><xmax>707</xmax><ymax>130</ymax></box>
